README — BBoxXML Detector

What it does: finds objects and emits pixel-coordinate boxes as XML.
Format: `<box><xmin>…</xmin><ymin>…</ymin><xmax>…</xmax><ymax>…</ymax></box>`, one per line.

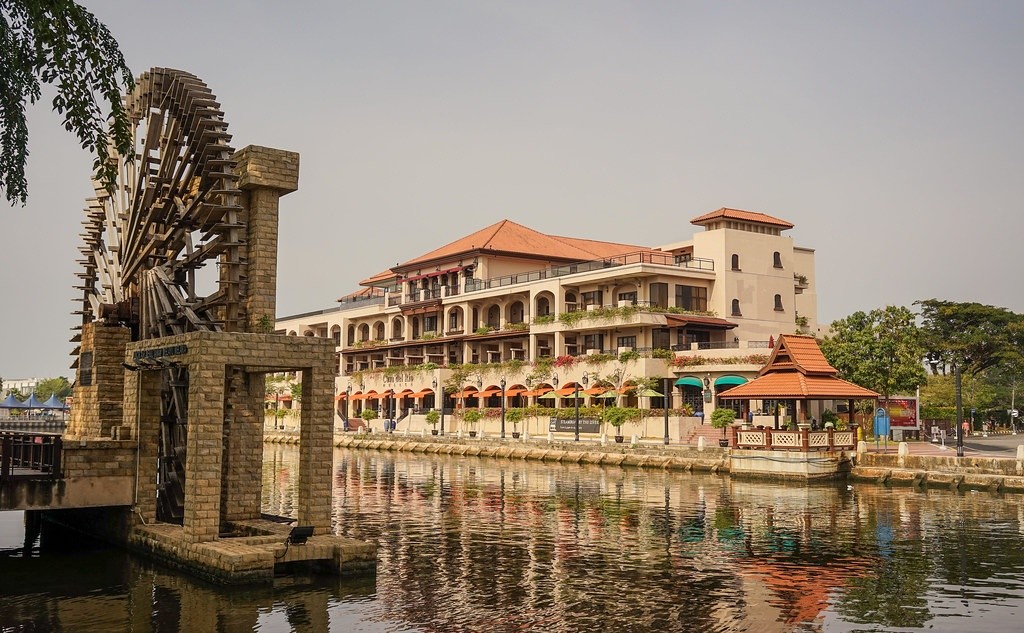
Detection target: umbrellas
<box><xmin>769</xmin><ymin>335</ymin><xmax>775</xmax><ymax>348</ymax></box>
<box><xmin>539</xmin><ymin>389</ymin><xmax>628</xmax><ymax>408</ymax></box>
<box><xmin>634</xmin><ymin>389</ymin><xmax>665</xmax><ymax>397</ymax></box>
<box><xmin>0</xmin><ymin>391</ymin><xmax>70</xmax><ymax>420</ymax></box>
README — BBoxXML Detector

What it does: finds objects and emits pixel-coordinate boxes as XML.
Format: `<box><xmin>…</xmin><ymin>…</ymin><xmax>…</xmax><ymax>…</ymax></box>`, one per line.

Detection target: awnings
<box><xmin>714</xmin><ymin>375</ymin><xmax>749</xmax><ymax>388</ymax></box>
<box><xmin>335</xmin><ymin>391</ymin><xmax>434</xmax><ymax>400</ymax></box>
<box><xmin>675</xmin><ymin>377</ymin><xmax>703</xmax><ymax>390</ymax></box>
<box><xmin>450</xmin><ymin>386</ymin><xmax>639</xmax><ymax>398</ymax></box>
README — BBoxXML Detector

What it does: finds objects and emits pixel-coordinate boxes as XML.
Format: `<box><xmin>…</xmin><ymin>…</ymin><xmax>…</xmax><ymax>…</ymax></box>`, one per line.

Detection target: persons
<box><xmin>982</xmin><ymin>416</ymin><xmax>999</xmax><ymax>433</ymax></box>
<box><xmin>811</xmin><ymin>419</ymin><xmax>819</xmax><ymax>432</ymax></box>
<box><xmin>961</xmin><ymin>420</ymin><xmax>970</xmax><ymax>437</ymax></box>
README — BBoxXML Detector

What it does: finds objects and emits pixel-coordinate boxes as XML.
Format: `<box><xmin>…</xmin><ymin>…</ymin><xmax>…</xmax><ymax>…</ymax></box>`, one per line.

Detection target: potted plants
<box><xmin>278</xmin><ymin>409</ymin><xmax>285</xmax><ymax>429</ymax></box>
<box><xmin>606</xmin><ymin>408</ymin><xmax>628</xmax><ymax>443</ymax></box>
<box><xmin>505</xmin><ymin>408</ymin><xmax>522</xmax><ymax>438</ymax></box>
<box><xmin>362</xmin><ymin>409</ymin><xmax>376</xmax><ymax>432</ymax></box>
<box><xmin>425</xmin><ymin>411</ymin><xmax>440</xmax><ymax>435</ymax></box>
<box><xmin>464</xmin><ymin>408</ymin><xmax>479</xmax><ymax>437</ymax></box>
<box><xmin>710</xmin><ymin>408</ymin><xmax>736</xmax><ymax>446</ymax></box>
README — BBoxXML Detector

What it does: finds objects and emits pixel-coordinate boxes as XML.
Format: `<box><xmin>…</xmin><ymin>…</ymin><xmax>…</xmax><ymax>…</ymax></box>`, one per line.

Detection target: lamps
<box><xmin>477</xmin><ymin>376</ymin><xmax>482</xmax><ymax>387</ymax></box>
<box><xmin>553</xmin><ymin>372</ymin><xmax>558</xmax><ymax>386</ymax></box>
<box><xmin>432</xmin><ymin>376</ymin><xmax>437</xmax><ymax>389</ymax></box>
<box><xmin>359</xmin><ymin>380</ymin><xmax>365</xmax><ymax>390</ymax></box>
<box><xmin>347</xmin><ymin>381</ymin><xmax>351</xmax><ymax>392</ymax></box>
<box><xmin>525</xmin><ymin>372</ymin><xmax>531</xmax><ymax>386</ymax></box>
<box><xmin>500</xmin><ymin>375</ymin><xmax>506</xmax><ymax>385</ymax></box>
<box><xmin>121</xmin><ymin>355</ymin><xmax>176</xmax><ymax>371</ymax></box>
<box><xmin>704</xmin><ymin>376</ymin><xmax>709</xmax><ymax>389</ymax></box>
<box><xmin>289</xmin><ymin>526</ymin><xmax>315</xmax><ymax>544</ymax></box>
<box><xmin>614</xmin><ymin>369</ymin><xmax>619</xmax><ymax>384</ymax></box>
<box><xmin>582</xmin><ymin>371</ymin><xmax>588</xmax><ymax>384</ymax></box>
